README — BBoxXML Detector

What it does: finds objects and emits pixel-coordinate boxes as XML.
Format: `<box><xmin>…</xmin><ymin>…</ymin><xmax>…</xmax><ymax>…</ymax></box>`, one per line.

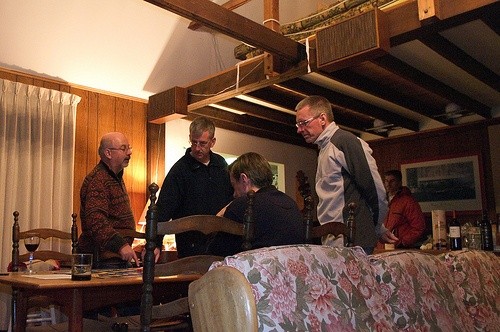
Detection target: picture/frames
<box><xmin>397</xmin><ymin>150</ymin><xmax>488</xmax><ymax>218</ymax></box>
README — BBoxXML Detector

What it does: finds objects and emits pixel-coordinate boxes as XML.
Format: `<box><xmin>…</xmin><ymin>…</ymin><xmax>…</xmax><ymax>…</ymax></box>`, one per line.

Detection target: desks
<box><xmin>0</xmin><ymin>266</ymin><xmax>204</xmax><ymax>332</ymax></box>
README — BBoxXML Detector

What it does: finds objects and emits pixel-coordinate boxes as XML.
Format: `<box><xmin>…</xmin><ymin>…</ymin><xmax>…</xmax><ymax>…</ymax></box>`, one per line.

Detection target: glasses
<box><xmin>295</xmin><ymin>112</ymin><xmax>323</xmax><ymax>128</ymax></box>
<box><xmin>108</xmin><ymin>144</ymin><xmax>132</xmax><ymax>152</ymax></box>
<box><xmin>188</xmin><ymin>138</ymin><xmax>212</xmax><ymax>147</ymax></box>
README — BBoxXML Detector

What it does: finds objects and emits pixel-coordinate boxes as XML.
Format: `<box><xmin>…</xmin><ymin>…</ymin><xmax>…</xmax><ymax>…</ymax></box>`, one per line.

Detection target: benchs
<box><xmin>187</xmin><ymin>244</ymin><xmax>500</xmax><ymax>332</ymax></box>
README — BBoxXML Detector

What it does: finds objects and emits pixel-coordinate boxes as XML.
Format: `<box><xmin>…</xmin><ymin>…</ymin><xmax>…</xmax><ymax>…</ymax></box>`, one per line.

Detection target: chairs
<box><xmin>303</xmin><ymin>195</ymin><xmax>357</xmax><ymax>248</ymax></box>
<box><xmin>11</xmin><ymin>211</ymin><xmax>78</xmax><ymax>332</ymax></box>
<box><xmin>96</xmin><ymin>183</ymin><xmax>257</xmax><ymax>332</ymax></box>
<box><xmin>115</xmin><ymin>229</ymin><xmax>164</xmax><ymax>269</ymax></box>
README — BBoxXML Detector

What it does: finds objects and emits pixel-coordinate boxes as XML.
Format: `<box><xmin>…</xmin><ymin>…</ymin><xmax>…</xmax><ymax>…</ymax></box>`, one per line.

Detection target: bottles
<box><xmin>481</xmin><ymin>210</ymin><xmax>494</xmax><ymax>251</ymax></box>
<box><xmin>447</xmin><ymin>209</ymin><xmax>462</xmax><ymax>251</ymax></box>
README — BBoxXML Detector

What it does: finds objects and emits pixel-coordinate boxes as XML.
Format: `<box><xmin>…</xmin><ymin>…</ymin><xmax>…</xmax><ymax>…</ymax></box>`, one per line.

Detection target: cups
<box><xmin>467</xmin><ymin>233</ymin><xmax>480</xmax><ymax>250</ymax></box>
<box><xmin>71</xmin><ymin>253</ymin><xmax>93</xmax><ymax>281</ymax></box>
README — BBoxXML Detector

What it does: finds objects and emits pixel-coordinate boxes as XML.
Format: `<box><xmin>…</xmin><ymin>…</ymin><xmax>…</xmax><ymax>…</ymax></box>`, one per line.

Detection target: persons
<box><xmin>376</xmin><ymin>169</ymin><xmax>425</xmax><ymax>250</ymax></box>
<box><xmin>206</xmin><ymin>152</ymin><xmax>306</xmax><ymax>257</ymax></box>
<box><xmin>141</xmin><ymin>117</ymin><xmax>234</xmax><ymax>263</ymax></box>
<box><xmin>76</xmin><ymin>131</ymin><xmax>141</xmax><ymax>320</ymax></box>
<box><xmin>295</xmin><ymin>96</ymin><xmax>389</xmax><ymax>255</ymax></box>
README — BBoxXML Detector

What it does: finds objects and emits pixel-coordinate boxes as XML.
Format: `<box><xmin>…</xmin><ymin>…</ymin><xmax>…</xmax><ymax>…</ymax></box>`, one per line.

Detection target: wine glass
<box><xmin>23</xmin><ymin>233</ymin><xmax>41</xmax><ymax>275</ymax></box>
